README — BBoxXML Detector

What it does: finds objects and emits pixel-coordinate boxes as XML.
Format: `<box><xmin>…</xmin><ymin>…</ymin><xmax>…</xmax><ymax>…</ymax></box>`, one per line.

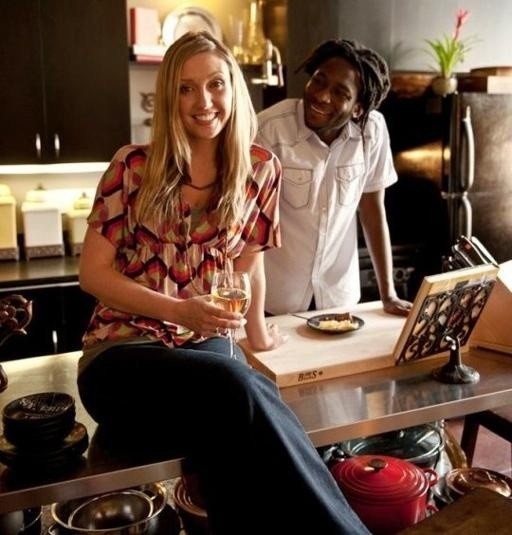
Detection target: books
<box><xmin>392</xmin><ymin>260</ymin><xmax>499</xmax><ymax>362</ymax></box>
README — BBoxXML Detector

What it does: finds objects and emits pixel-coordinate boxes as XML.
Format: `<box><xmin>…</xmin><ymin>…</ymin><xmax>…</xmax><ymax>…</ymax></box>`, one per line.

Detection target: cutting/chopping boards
<box><xmin>230</xmin><ymin>298</ymin><xmax>469</xmax><ymax>388</ymax></box>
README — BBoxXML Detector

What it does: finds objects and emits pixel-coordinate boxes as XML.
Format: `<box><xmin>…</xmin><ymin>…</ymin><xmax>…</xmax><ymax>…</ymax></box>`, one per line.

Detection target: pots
<box><xmin>333</xmin><ymin>424</ymin><xmax>510</xmax><ymax>531</ymax></box>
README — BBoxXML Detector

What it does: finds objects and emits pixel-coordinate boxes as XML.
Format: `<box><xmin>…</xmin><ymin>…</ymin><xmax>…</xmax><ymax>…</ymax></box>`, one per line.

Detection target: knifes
<box><xmin>442</xmin><ymin>235</ymin><xmax>499</xmax><ymax>272</ymax></box>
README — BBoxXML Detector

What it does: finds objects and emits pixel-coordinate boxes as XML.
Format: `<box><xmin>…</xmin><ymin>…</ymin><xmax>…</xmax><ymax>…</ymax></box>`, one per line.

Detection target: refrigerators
<box><xmin>379</xmin><ymin>93</ymin><xmax>512</xmax><ymax>265</ymax></box>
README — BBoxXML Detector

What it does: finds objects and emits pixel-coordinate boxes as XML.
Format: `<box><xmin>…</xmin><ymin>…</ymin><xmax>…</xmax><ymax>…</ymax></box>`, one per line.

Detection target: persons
<box><xmin>254</xmin><ymin>36</ymin><xmax>415</xmax><ymax>316</ymax></box>
<box><xmin>78</xmin><ymin>30</ymin><xmax>370</xmax><ymax>535</ymax></box>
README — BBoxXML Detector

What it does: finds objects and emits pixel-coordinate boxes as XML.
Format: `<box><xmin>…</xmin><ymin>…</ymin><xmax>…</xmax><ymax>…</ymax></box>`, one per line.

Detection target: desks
<box><xmin>0</xmin><ymin>306</ymin><xmax>511</xmax><ymax>534</ymax></box>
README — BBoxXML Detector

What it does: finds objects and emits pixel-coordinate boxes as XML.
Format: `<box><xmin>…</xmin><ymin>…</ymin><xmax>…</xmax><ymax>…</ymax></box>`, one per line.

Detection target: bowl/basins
<box><xmin>1</xmin><ymin>477</ymin><xmax>207</xmax><ymax>535</ymax></box>
<box><xmin>3</xmin><ymin>393</ymin><xmax>78</xmax><ymax>452</ymax></box>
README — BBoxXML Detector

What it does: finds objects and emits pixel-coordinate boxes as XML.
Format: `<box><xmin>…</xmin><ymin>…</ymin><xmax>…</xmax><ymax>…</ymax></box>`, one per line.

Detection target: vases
<box><xmin>429</xmin><ymin>76</ymin><xmax>460</xmax><ymax>98</ymax></box>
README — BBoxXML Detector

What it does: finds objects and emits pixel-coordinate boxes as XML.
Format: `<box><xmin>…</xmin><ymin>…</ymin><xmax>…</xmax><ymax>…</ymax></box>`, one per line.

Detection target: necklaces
<box><xmin>188</xmin><ymin>180</ymin><xmax>215</xmax><ymax>191</ymax></box>
<box><xmin>179</xmin><ymin>185</ymin><xmax>227</xmax><ymax>297</ymax></box>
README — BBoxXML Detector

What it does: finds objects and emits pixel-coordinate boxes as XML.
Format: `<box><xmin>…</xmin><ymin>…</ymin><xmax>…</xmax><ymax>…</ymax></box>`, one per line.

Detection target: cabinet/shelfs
<box><xmin>1</xmin><ymin>278</ymin><xmax>97</xmax><ymax>361</ymax></box>
<box><xmin>1</xmin><ymin>0</ymin><xmax>134</xmax><ymax>178</ymax></box>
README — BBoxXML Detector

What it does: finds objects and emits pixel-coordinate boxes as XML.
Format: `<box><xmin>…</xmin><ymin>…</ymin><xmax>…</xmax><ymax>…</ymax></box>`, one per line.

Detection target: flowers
<box><xmin>417</xmin><ymin>6</ymin><xmax>490</xmax><ymax>78</ymax></box>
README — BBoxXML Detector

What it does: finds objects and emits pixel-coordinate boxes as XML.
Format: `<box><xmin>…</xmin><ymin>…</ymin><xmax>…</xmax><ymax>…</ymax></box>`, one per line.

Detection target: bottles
<box><xmin>230</xmin><ymin>0</ymin><xmax>265</xmax><ymax>64</ymax></box>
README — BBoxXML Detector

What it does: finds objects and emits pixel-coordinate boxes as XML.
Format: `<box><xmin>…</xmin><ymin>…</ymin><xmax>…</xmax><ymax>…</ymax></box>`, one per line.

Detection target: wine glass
<box><xmin>213</xmin><ymin>272</ymin><xmax>255</xmax><ymax>370</ymax></box>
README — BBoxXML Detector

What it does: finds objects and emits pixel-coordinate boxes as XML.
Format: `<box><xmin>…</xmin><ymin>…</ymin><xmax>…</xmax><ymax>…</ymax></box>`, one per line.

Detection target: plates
<box><xmin>162</xmin><ymin>6</ymin><xmax>223</xmax><ymax>47</ymax></box>
<box><xmin>307</xmin><ymin>314</ymin><xmax>364</xmax><ymax>335</ymax></box>
<box><xmin>1</xmin><ymin>420</ymin><xmax>90</xmax><ymax>471</ymax></box>
